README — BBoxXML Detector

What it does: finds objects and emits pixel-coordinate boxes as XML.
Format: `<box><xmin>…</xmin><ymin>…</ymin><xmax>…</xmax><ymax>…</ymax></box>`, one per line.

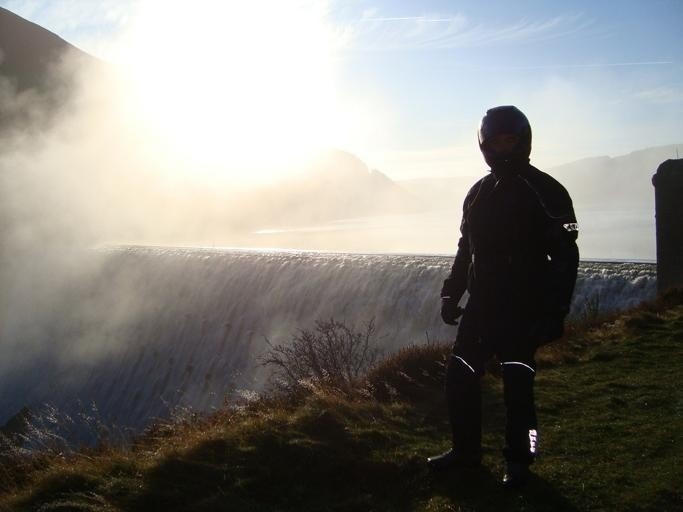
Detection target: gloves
<box><xmin>440</xmin><ymin>280</ymin><xmax>464</xmax><ymax>325</ymax></box>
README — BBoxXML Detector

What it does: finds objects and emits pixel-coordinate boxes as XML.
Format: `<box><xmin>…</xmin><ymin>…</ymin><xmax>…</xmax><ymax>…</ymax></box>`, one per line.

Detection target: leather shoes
<box><xmin>497</xmin><ymin>462</ymin><xmax>530</xmax><ymax>488</ymax></box>
<box><xmin>427</xmin><ymin>448</ymin><xmax>480</xmax><ymax>468</ymax></box>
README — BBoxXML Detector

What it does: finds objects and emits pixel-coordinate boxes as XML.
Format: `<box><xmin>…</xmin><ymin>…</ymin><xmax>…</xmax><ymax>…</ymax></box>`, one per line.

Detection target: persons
<box><xmin>428</xmin><ymin>106</ymin><xmax>580</xmax><ymax>495</ymax></box>
